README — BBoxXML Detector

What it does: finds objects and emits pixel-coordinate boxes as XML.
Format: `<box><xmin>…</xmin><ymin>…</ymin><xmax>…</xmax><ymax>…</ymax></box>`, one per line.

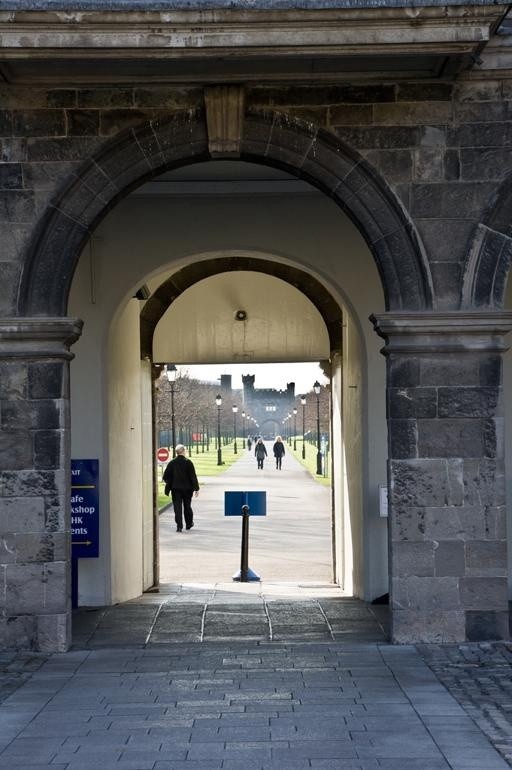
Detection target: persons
<box><xmin>255</xmin><ymin>439</ymin><xmax>267</xmax><ymax>469</ymax></box>
<box><xmin>163</xmin><ymin>444</ymin><xmax>199</xmax><ymax>533</ymax></box>
<box><xmin>273</xmin><ymin>436</ymin><xmax>285</xmax><ymax>470</ymax></box>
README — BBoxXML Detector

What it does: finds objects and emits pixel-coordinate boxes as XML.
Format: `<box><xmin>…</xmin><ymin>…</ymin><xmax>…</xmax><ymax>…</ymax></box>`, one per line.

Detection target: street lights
<box><xmin>232</xmin><ymin>404</ymin><xmax>260</xmax><ymax>454</ymax></box>
<box><xmin>282</xmin><ymin>395</ymin><xmax>307</xmax><ymax>459</ymax></box>
<box><xmin>214</xmin><ymin>394</ymin><xmax>223</xmax><ymax>466</ymax></box>
<box><xmin>165</xmin><ymin>364</ymin><xmax>180</xmax><ymax>458</ymax></box>
<box><xmin>313</xmin><ymin>380</ymin><xmax>322</xmax><ymax>475</ymax></box>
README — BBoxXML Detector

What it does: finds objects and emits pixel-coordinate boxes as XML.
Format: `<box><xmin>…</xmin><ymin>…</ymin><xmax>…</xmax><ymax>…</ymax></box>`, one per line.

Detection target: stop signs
<box><xmin>156</xmin><ymin>448</ymin><xmax>169</xmax><ymax>464</ymax></box>
